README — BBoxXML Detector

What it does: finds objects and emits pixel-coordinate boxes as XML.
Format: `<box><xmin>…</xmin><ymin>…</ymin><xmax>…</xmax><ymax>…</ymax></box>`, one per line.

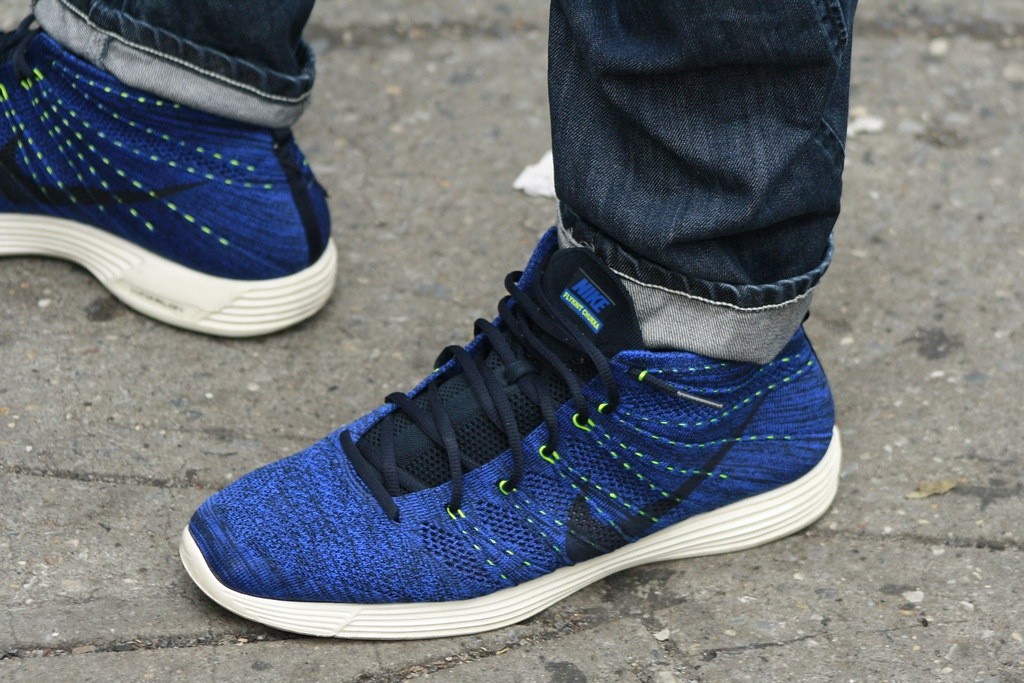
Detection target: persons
<box><xmin>0</xmin><ymin>0</ymin><xmax>856</xmax><ymax>641</ymax></box>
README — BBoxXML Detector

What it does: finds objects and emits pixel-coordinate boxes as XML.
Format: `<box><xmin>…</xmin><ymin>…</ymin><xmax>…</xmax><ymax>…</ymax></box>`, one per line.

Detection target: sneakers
<box><xmin>179</xmin><ymin>226</ymin><xmax>842</xmax><ymax>640</ymax></box>
<box><xmin>0</xmin><ymin>12</ymin><xmax>338</xmax><ymax>338</ymax></box>
